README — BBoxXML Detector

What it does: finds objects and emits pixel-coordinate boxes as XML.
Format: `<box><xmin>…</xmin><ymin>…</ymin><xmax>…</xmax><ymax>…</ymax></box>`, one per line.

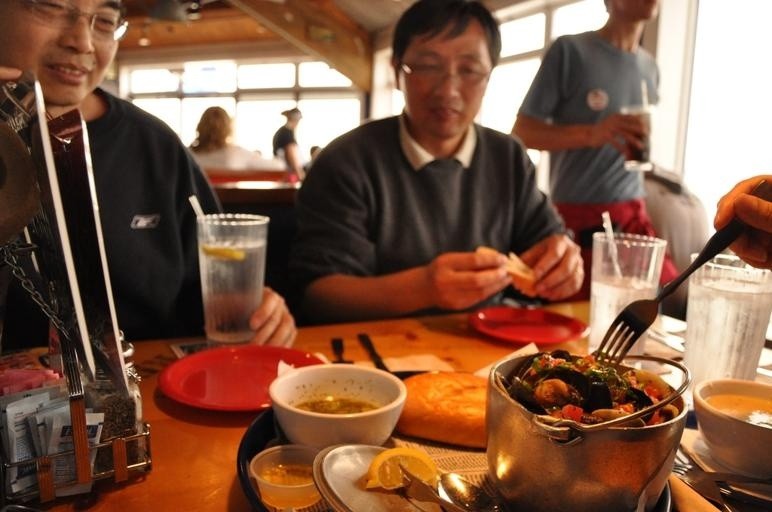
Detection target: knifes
<box><xmin>356</xmin><ymin>332</ymin><xmax>391</xmax><ymax>371</ymax></box>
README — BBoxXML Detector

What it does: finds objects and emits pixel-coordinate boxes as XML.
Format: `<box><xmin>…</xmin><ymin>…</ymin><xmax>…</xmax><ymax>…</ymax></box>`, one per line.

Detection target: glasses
<box><xmin>32</xmin><ymin>0</ymin><xmax>128</xmax><ymax>40</ymax></box>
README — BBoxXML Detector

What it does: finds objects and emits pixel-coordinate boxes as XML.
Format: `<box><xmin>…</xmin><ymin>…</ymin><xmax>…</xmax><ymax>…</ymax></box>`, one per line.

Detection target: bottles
<box><xmin>81</xmin><ymin>340</ymin><xmax>144</xmax><ymax>483</ymax></box>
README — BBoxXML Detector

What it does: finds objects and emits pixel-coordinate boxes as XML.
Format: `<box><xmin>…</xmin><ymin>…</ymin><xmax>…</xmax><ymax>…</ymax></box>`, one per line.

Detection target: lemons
<box><xmin>367</xmin><ymin>445</ymin><xmax>438</xmax><ymax>492</ymax></box>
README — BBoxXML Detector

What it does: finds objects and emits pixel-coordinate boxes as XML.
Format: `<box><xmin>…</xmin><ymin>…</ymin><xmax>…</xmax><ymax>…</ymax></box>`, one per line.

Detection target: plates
<box><xmin>469</xmin><ymin>307</ymin><xmax>588</xmax><ymax>347</ymax></box>
<box><xmin>160</xmin><ymin>344</ymin><xmax>324</xmax><ymax>413</ymax></box>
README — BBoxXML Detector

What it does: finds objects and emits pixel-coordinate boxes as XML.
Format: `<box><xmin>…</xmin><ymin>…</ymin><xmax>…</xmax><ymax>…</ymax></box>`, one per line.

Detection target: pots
<box><xmin>690</xmin><ymin>377</ymin><xmax>772</xmax><ymax>477</ymax></box>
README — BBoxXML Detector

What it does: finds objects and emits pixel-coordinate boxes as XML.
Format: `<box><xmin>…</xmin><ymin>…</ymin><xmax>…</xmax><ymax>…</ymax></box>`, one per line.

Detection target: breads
<box><xmin>397</xmin><ymin>371</ymin><xmax>489</xmax><ymax>447</ymax></box>
<box><xmin>475</xmin><ymin>245</ymin><xmax>539</xmax><ymax>299</ymax></box>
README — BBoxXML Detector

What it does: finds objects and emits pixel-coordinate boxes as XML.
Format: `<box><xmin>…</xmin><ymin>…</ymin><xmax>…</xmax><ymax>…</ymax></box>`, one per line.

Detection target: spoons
<box><xmin>437</xmin><ymin>471</ymin><xmax>497</xmax><ymax>511</ymax></box>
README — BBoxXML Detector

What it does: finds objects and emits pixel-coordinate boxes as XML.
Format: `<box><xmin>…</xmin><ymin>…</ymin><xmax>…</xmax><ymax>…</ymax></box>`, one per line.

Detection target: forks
<box><xmin>594</xmin><ymin>178</ymin><xmax>771</xmax><ymax>372</ymax></box>
<box><xmin>397</xmin><ymin>462</ymin><xmax>462</xmax><ymax>510</ymax></box>
<box><xmin>671</xmin><ymin>463</ymin><xmax>738</xmax><ymax>512</ymax></box>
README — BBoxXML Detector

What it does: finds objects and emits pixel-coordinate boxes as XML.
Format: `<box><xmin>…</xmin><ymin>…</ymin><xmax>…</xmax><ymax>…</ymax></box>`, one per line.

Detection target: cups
<box><xmin>622</xmin><ymin>104</ymin><xmax>655</xmax><ymax>172</ymax></box>
<box><xmin>195</xmin><ymin>212</ymin><xmax>272</xmax><ymax>340</ymax></box>
<box><xmin>588</xmin><ymin>231</ymin><xmax>668</xmax><ymax>371</ymax></box>
<box><xmin>685</xmin><ymin>252</ymin><xmax>771</xmax><ymax>405</ymax></box>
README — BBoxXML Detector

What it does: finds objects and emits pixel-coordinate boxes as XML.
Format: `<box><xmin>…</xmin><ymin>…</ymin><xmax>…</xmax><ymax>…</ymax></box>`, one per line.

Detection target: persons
<box><xmin>1</xmin><ymin>0</ymin><xmax>297</xmax><ymax>348</ymax></box>
<box><xmin>301</xmin><ymin>147</ymin><xmax>323</xmax><ymax>177</ymax></box>
<box><xmin>715</xmin><ymin>173</ymin><xmax>772</xmax><ymax>270</ymax></box>
<box><xmin>186</xmin><ymin>107</ymin><xmax>286</xmax><ymax>173</ymax></box>
<box><xmin>273</xmin><ymin>108</ymin><xmax>304</xmax><ymax>180</ymax></box>
<box><xmin>512</xmin><ymin>0</ymin><xmax>685</xmax><ymax>320</ymax></box>
<box><xmin>260</xmin><ymin>0</ymin><xmax>587</xmax><ymax>326</ymax></box>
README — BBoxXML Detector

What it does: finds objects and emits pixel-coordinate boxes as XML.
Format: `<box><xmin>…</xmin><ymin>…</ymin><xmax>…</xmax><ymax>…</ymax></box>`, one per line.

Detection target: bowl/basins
<box><xmin>268</xmin><ymin>362</ymin><xmax>407</xmax><ymax>448</ymax></box>
<box><xmin>485</xmin><ymin>350</ymin><xmax>689</xmax><ymax>511</ymax></box>
<box><xmin>248</xmin><ymin>444</ymin><xmax>321</xmax><ymax>509</ymax></box>
